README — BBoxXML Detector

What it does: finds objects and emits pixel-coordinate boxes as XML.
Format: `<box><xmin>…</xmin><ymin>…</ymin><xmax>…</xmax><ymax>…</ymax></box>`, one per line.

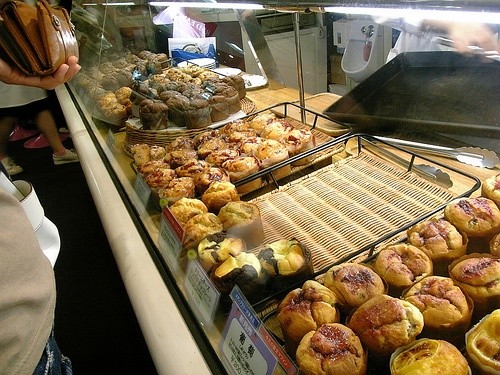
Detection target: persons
<box><xmin>384</xmin><ymin>12</ymin><xmax>499</xmax><ymax>61</ymax></box>
<box><xmin>0</xmin><ymin>0</ymin><xmax>82</xmax><ymax>375</ymax></box>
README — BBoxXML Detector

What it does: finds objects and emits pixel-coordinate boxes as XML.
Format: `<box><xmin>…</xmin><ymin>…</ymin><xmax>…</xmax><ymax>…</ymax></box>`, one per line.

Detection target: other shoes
<box><xmin>7</xmin><ymin>125</ymin><xmax>36</xmax><ymax>141</ymax></box>
<box><xmin>24</xmin><ymin>133</ymin><xmax>68</xmax><ymax>149</ymax></box>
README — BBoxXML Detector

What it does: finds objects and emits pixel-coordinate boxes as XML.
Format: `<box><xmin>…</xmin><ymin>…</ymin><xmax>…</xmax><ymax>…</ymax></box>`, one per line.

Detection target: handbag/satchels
<box><xmin>0</xmin><ymin>0</ymin><xmax>80</xmax><ymax>75</ymax></box>
<box><xmin>0</xmin><ymin>172</ymin><xmax>60</xmax><ymax>270</ymax></box>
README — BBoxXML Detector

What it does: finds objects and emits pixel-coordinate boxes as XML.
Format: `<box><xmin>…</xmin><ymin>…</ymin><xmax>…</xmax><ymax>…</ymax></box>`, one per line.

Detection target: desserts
<box><xmin>74</xmin><ymin>51</ymin><xmax>247</xmax><ymax>129</ymax></box>
<box><xmin>277</xmin><ymin>173</ymin><xmax>500</xmax><ymax>375</ymax></box>
<box><xmin>133</xmin><ymin>110</ymin><xmax>313</xmax><ymax>202</ymax></box>
<box><xmin>167</xmin><ymin>180</ymin><xmax>315</xmax><ymax>311</ymax></box>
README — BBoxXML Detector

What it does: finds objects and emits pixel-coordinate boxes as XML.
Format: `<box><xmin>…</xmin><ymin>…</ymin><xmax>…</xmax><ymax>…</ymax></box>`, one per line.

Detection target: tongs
<box><xmin>365</xmin><ymin>134</ymin><xmax>500</xmax><ymax>190</ymax></box>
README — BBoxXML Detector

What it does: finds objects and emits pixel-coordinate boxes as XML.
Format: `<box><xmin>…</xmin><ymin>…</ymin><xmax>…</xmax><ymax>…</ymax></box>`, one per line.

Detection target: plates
<box><xmin>210</xmin><ymin>68</ymin><xmax>241</xmax><ymax>79</ymax></box>
<box><xmin>241</xmin><ymin>74</ymin><xmax>267</xmax><ymax>90</ymax></box>
<box><xmin>177</xmin><ymin>58</ymin><xmax>217</xmax><ymax>70</ymax></box>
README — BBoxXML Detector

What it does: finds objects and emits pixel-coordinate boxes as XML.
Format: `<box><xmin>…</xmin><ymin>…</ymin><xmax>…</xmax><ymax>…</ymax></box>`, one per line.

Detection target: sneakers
<box><xmin>52</xmin><ymin>148</ymin><xmax>79</xmax><ymax>164</ymax></box>
<box><xmin>1</xmin><ymin>156</ymin><xmax>23</xmax><ymax>175</ymax></box>
